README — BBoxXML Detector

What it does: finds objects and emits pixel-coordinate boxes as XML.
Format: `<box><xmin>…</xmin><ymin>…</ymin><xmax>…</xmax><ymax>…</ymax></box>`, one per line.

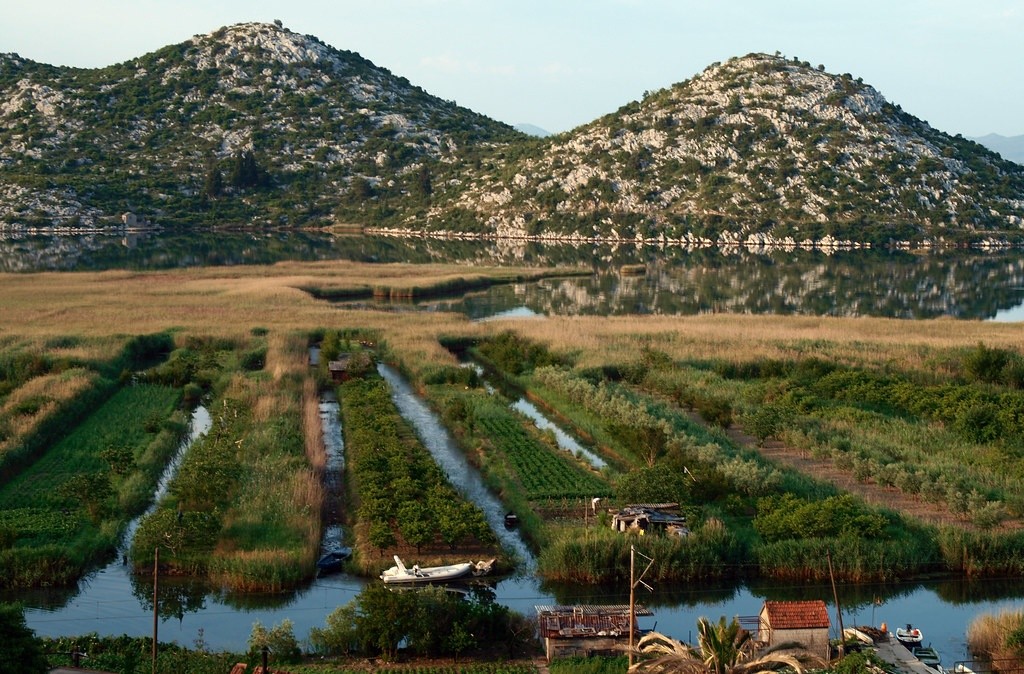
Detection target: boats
<box><xmin>912</xmin><ymin>646</ymin><xmax>941</xmax><ymax>667</ymax></box>
<box><xmin>380</xmin><ymin>555</ymin><xmax>471</xmax><ymax>583</ymax></box>
<box><xmin>937</xmin><ymin>663</ymin><xmax>977</xmax><ymax>674</ymax></box>
<box><xmin>504</xmin><ymin>510</ymin><xmax>517</xmax><ymax>527</ymax></box>
<box><xmin>895</xmin><ymin>624</ymin><xmax>923</xmax><ymax>643</ymax></box>
<box><xmin>317</xmin><ymin>547</ymin><xmax>353</xmax><ymax>573</ymax></box>
